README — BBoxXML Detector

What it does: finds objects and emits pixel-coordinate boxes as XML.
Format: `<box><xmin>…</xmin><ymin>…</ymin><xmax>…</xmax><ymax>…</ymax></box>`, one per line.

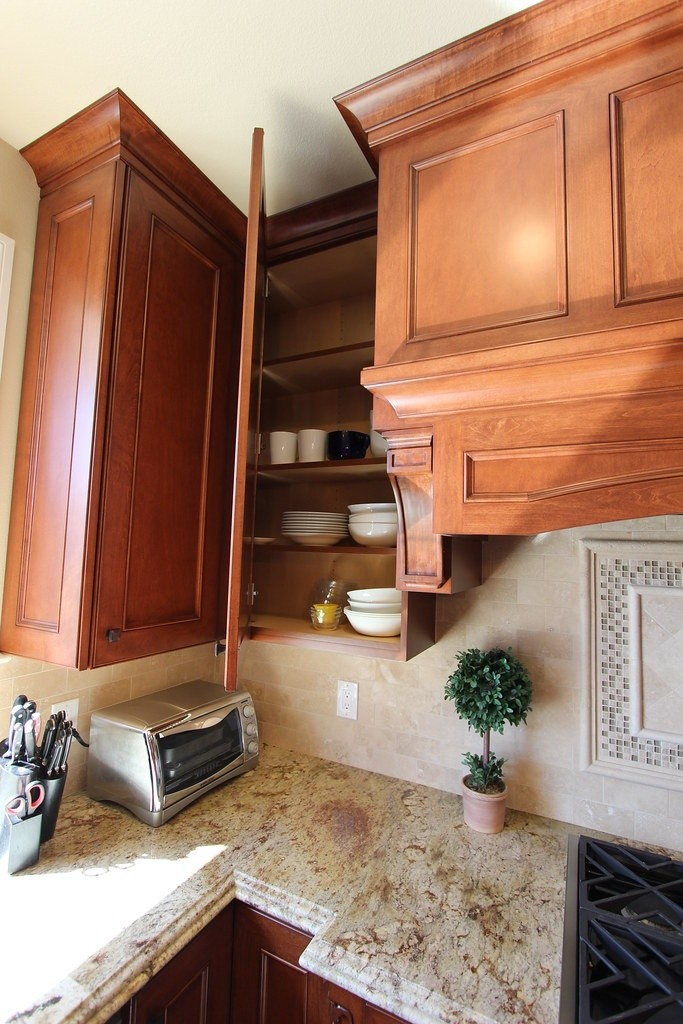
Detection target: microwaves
<box><xmin>85</xmin><ymin>679</ymin><xmax>261</xmax><ymax>830</ymax></box>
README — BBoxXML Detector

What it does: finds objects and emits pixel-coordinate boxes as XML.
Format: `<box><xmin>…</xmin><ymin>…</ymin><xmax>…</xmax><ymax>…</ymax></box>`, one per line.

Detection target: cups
<box><xmin>297</xmin><ymin>429</ymin><xmax>328</xmax><ymax>462</ymax></box>
<box><xmin>270</xmin><ymin>432</ymin><xmax>297</xmax><ymax>465</ymax></box>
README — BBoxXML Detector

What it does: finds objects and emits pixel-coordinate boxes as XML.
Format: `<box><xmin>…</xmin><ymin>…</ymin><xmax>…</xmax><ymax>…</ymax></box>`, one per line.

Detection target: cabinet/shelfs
<box><xmin>0</xmin><ymin>143</ymin><xmax>247</xmax><ymax>674</ymax></box>
<box><xmin>358</xmin><ymin>0</ymin><xmax>683</xmax><ymax>597</ymax></box>
<box><xmin>224</xmin><ymin>128</ymin><xmax>411</xmax><ymax>696</ymax></box>
<box><xmin>233</xmin><ymin>898</ymin><xmax>378</xmax><ymax>1024</ymax></box>
<box><xmin>125</xmin><ymin>899</ymin><xmax>234</xmax><ymax>1023</ymax></box>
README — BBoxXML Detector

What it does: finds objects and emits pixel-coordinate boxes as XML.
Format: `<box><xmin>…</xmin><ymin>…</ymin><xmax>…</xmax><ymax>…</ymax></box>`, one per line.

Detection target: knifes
<box><xmin>0</xmin><ymin>692</ymin><xmax>94</xmax><ymax>779</ymax></box>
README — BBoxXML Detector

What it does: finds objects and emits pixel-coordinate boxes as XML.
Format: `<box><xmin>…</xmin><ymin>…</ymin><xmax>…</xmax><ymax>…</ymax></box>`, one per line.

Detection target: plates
<box><xmin>280</xmin><ymin>511</ymin><xmax>349</xmax><ymax>547</ymax></box>
<box><xmin>254</xmin><ymin>537</ymin><xmax>276</xmax><ymax>545</ymax></box>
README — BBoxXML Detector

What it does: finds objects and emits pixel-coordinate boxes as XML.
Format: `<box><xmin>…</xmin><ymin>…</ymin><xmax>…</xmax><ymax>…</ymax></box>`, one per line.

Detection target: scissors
<box><xmin>5</xmin><ymin>780</ymin><xmax>46</xmax><ymax>819</ymax></box>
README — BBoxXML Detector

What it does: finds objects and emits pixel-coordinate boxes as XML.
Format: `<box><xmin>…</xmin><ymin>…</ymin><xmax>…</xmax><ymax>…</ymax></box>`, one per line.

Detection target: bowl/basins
<box><xmin>348</xmin><ymin>503</ymin><xmax>398</xmax><ymax>549</ymax></box>
<box><xmin>367</xmin><ymin>410</ymin><xmax>388</xmax><ymax>457</ymax></box>
<box><xmin>308</xmin><ymin>577</ymin><xmax>401</xmax><ymax>639</ymax></box>
<box><xmin>326</xmin><ymin>431</ymin><xmax>370</xmax><ymax>460</ymax></box>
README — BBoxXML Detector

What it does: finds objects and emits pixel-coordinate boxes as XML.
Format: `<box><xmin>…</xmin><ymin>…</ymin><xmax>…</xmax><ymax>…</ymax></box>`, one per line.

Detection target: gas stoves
<box><xmin>555</xmin><ymin>833</ymin><xmax>683</xmax><ymax>1024</ymax></box>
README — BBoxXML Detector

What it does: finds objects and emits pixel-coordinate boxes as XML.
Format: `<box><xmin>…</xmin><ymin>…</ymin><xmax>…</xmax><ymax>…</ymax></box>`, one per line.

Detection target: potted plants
<box><xmin>442</xmin><ymin>647</ymin><xmax>536</xmax><ymax>834</ymax></box>
